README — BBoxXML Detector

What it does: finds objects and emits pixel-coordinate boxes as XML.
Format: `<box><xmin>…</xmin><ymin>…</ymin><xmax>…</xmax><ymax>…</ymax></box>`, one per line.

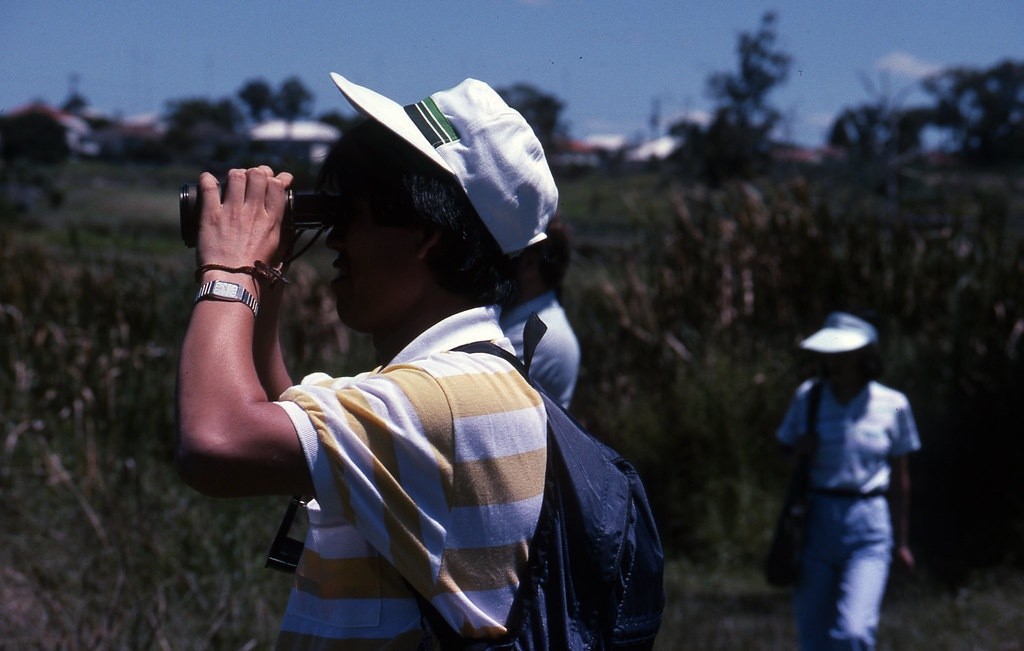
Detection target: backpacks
<box><xmin>447</xmin><ymin>312</ymin><xmax>665</xmax><ymax>650</ymax></box>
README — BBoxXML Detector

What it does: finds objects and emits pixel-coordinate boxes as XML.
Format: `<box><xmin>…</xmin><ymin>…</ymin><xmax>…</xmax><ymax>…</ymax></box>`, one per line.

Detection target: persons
<box><xmin>475</xmin><ymin>227</ymin><xmax>578</xmax><ymax>411</ymax></box>
<box><xmin>775</xmin><ymin>310</ymin><xmax>921</xmax><ymax>651</ymax></box>
<box><xmin>175</xmin><ymin>72</ymin><xmax>559</xmax><ymax>651</ymax></box>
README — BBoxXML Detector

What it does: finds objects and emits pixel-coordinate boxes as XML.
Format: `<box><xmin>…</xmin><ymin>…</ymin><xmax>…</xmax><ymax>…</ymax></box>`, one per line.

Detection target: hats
<box><xmin>327</xmin><ymin>69</ymin><xmax>560</xmax><ymax>257</ymax></box>
<box><xmin>802</xmin><ymin>312</ymin><xmax>880</xmax><ymax>352</ymax></box>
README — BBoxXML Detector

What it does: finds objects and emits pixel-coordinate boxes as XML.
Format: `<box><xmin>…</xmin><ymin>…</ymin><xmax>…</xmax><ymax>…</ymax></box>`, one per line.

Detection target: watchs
<box><xmin>192</xmin><ymin>280</ymin><xmax>260</xmax><ymax>317</ymax></box>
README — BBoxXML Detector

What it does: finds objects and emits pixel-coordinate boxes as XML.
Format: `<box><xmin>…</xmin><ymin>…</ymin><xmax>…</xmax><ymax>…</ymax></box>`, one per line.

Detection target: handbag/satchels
<box><xmin>759</xmin><ymin>379</ymin><xmax>828</xmax><ymax>589</ymax></box>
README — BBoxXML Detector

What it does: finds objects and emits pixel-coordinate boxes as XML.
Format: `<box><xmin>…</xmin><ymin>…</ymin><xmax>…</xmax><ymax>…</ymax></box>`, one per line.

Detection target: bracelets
<box><xmin>193</xmin><ymin>258</ymin><xmax>289</xmax><ymax>291</ymax></box>
